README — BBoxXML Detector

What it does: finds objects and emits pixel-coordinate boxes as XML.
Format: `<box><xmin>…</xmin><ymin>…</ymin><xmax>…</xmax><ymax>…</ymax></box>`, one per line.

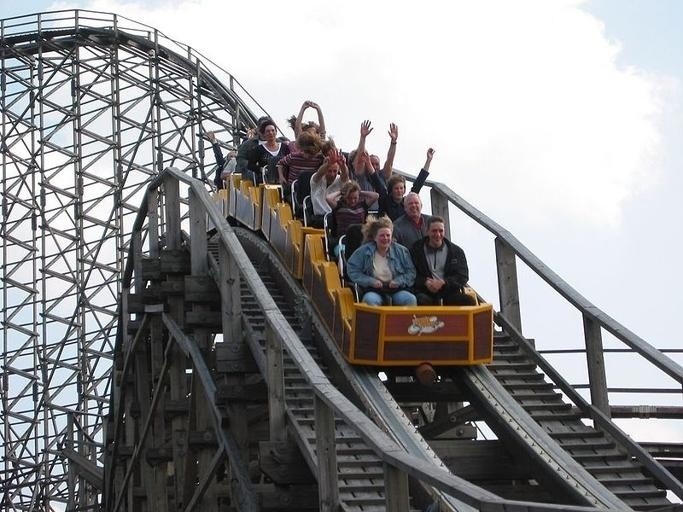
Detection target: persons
<box><xmin>310</xmin><ymin>152</ymin><xmax>353</xmax><ymax>227</ymax></box>
<box><xmin>392</xmin><ymin>192</ymin><xmax>450</xmax><ymax>254</ymax></box>
<box><xmin>326</xmin><ymin>182</ymin><xmax>380</xmax><ymax>246</ymax></box>
<box><xmin>412</xmin><ymin>217</ymin><xmax>475</xmax><ymax>306</ymax></box>
<box><xmin>208</xmin><ymin>116</ymin><xmax>406</xmax><ymax>212</ymax></box>
<box><xmin>347</xmin><ymin>216</ymin><xmax>416</xmax><ymax>308</ymax></box>
<box><xmin>360</xmin><ymin>147</ymin><xmax>436</xmax><ymax>221</ymax></box>
